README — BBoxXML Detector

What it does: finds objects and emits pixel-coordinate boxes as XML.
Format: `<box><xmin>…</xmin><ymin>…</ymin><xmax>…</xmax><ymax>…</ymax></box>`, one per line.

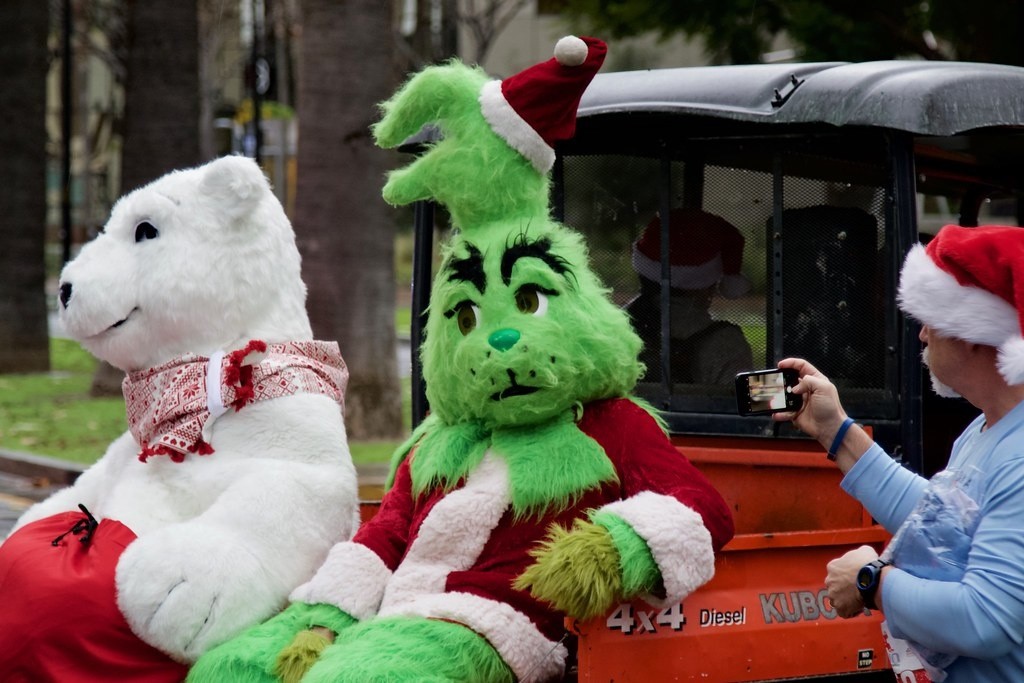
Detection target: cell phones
<box><xmin>735</xmin><ymin>368</ymin><xmax>804</xmax><ymax>416</ymax></box>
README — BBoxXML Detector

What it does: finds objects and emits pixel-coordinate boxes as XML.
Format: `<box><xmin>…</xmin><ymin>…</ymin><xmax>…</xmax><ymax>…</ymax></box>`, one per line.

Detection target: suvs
<box><xmin>394</xmin><ymin>58</ymin><xmax>1022</xmax><ymax>683</ymax></box>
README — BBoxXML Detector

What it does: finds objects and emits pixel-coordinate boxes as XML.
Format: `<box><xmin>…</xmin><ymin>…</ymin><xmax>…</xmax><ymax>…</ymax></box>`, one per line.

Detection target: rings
<box><xmin>828</xmin><ymin>598</ymin><xmax>833</xmax><ymax>611</ymax></box>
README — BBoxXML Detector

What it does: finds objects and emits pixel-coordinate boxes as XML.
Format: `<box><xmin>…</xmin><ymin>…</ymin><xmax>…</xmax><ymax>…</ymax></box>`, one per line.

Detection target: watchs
<box><xmin>853</xmin><ymin>554</ymin><xmax>893</xmax><ymax>613</ymax></box>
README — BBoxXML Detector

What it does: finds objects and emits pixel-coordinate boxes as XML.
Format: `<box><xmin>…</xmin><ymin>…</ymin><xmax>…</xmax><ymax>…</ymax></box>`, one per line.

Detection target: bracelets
<box><xmin>827</xmin><ymin>417</ymin><xmax>854</xmax><ymax>461</ymax></box>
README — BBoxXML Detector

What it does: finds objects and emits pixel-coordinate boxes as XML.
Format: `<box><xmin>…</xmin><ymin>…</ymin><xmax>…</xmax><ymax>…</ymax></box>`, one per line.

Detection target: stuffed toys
<box><xmin>184</xmin><ymin>34</ymin><xmax>736</xmax><ymax>683</ymax></box>
<box><xmin>0</xmin><ymin>155</ymin><xmax>361</xmax><ymax>683</ymax></box>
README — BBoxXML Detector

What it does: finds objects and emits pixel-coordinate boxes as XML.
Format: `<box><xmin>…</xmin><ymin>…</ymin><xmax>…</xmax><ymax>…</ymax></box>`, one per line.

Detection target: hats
<box><xmin>633</xmin><ymin>208</ymin><xmax>752</xmax><ymax>301</ymax></box>
<box><xmin>895</xmin><ymin>225</ymin><xmax>1024</xmax><ymax>385</ymax></box>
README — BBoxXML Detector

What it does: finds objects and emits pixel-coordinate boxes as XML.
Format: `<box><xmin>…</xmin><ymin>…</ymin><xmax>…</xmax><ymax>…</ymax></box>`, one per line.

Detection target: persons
<box><xmin>771</xmin><ymin>226</ymin><xmax>1024</xmax><ymax>683</ymax></box>
<box><xmin>614</xmin><ymin>207</ymin><xmax>757</xmax><ymax>418</ymax></box>
